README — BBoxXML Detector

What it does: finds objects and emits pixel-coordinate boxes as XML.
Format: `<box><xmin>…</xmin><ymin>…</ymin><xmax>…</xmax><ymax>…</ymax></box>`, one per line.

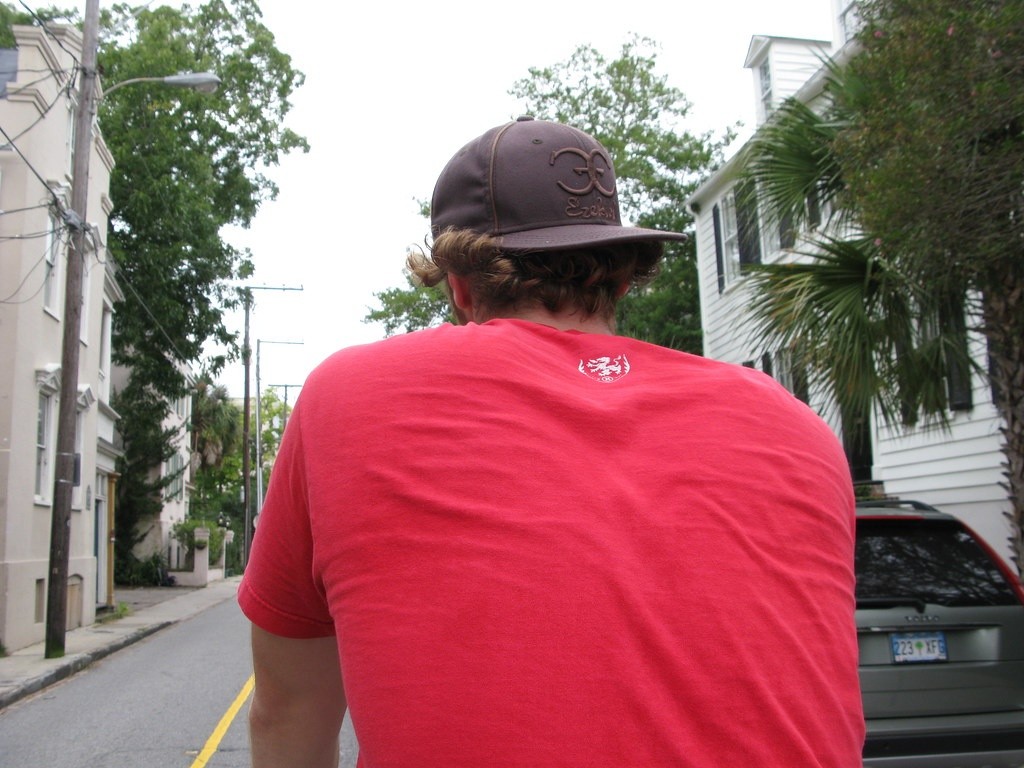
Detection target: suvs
<box><xmin>855</xmin><ymin>495</ymin><xmax>1024</xmax><ymax>768</ymax></box>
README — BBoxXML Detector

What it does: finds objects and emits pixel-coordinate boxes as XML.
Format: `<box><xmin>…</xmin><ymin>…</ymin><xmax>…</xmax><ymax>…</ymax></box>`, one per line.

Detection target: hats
<box><xmin>430</xmin><ymin>115</ymin><xmax>688</xmax><ymax>253</ymax></box>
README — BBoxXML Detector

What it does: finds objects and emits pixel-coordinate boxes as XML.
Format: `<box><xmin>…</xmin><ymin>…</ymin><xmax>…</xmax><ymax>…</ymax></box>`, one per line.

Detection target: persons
<box><xmin>238</xmin><ymin>117</ymin><xmax>866</xmax><ymax>768</ymax></box>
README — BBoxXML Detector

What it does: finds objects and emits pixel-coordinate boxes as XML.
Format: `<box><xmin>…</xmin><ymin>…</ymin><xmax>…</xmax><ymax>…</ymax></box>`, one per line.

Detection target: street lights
<box><xmin>44</xmin><ymin>73</ymin><xmax>221</xmax><ymax>659</ymax></box>
